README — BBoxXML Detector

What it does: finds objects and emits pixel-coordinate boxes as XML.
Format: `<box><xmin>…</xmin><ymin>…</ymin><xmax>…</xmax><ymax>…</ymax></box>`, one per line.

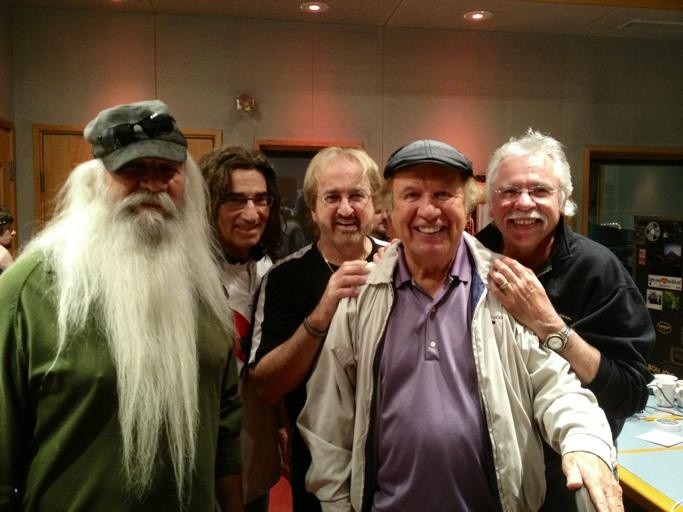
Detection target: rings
<box><xmin>500</xmin><ymin>279</ymin><xmax>509</xmax><ymax>289</ymax></box>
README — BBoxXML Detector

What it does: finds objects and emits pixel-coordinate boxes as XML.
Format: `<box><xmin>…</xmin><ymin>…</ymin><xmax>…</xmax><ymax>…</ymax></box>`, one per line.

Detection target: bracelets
<box><xmin>300</xmin><ymin>317</ymin><xmax>330</xmax><ymax>342</ymax></box>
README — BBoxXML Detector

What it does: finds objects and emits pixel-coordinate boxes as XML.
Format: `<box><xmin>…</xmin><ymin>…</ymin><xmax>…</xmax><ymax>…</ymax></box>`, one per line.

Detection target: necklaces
<box><xmin>316</xmin><ymin>237</ymin><xmax>371</xmax><ymax>277</ymax></box>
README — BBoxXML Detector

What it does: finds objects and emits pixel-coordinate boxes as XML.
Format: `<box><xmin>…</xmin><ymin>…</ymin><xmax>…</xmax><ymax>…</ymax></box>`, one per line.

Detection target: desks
<box><xmin>614</xmin><ymin>395</ymin><xmax>683</xmax><ymax>512</ymax></box>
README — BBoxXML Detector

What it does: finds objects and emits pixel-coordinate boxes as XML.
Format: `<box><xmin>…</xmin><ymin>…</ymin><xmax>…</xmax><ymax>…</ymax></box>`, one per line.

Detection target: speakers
<box><xmin>632</xmin><ymin>215</ymin><xmax>683</xmax><ymax>380</ymax></box>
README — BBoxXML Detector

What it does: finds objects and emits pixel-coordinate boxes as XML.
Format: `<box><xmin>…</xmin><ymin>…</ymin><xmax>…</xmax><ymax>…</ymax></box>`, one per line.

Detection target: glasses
<box><xmin>92</xmin><ymin>111</ymin><xmax>177</xmax><ymax>151</ymax></box>
<box><xmin>315</xmin><ymin>191</ymin><xmax>370</xmax><ymax>210</ymax></box>
<box><xmin>222</xmin><ymin>192</ymin><xmax>272</xmax><ymax>210</ymax></box>
<box><xmin>497</xmin><ymin>184</ymin><xmax>553</xmax><ymax>199</ymax></box>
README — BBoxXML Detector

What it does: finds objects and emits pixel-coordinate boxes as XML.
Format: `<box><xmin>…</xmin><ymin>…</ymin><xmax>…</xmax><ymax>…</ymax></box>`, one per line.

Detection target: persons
<box><xmin>473</xmin><ymin>130</ymin><xmax>655</xmax><ymax>511</ymax></box>
<box><xmin>243</xmin><ymin>146</ymin><xmax>395</xmax><ymax>511</ymax></box>
<box><xmin>0</xmin><ymin>99</ymin><xmax>244</xmax><ymax>509</ymax></box>
<box><xmin>370</xmin><ymin>206</ymin><xmax>392</xmax><ymax>242</ymax></box>
<box><xmin>294</xmin><ymin>140</ymin><xmax>627</xmax><ymax>511</ymax></box>
<box><xmin>294</xmin><ymin>189</ymin><xmax>321</xmax><ymax>241</ymax></box>
<box><xmin>193</xmin><ymin>142</ymin><xmax>293</xmax><ymax>511</ymax></box>
<box><xmin>0</xmin><ymin>204</ymin><xmax>16</xmax><ymax>273</ymax></box>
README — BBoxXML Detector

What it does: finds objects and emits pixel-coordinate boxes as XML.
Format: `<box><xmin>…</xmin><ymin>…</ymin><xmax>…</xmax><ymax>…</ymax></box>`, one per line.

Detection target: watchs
<box><xmin>537</xmin><ymin>321</ymin><xmax>574</xmax><ymax>354</ymax></box>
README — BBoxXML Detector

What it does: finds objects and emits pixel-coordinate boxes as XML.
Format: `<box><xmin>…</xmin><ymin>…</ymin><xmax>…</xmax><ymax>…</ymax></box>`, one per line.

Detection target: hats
<box><xmin>82</xmin><ymin>99</ymin><xmax>187</xmax><ymax>174</ymax></box>
<box><xmin>384</xmin><ymin>138</ymin><xmax>473</xmax><ymax>180</ymax></box>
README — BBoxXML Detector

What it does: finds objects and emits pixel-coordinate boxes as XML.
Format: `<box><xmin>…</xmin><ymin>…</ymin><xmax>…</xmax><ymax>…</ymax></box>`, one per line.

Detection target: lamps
<box><xmin>463</xmin><ymin>10</ymin><xmax>493</xmax><ymax>23</ymax></box>
<box><xmin>300</xmin><ymin>1</ymin><xmax>330</xmax><ymax>14</ymax></box>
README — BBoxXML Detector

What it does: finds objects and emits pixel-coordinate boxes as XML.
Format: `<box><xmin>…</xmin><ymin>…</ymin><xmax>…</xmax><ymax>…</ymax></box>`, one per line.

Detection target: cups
<box><xmin>647</xmin><ymin>372</ymin><xmax>682</xmax><ymax>408</ymax></box>
